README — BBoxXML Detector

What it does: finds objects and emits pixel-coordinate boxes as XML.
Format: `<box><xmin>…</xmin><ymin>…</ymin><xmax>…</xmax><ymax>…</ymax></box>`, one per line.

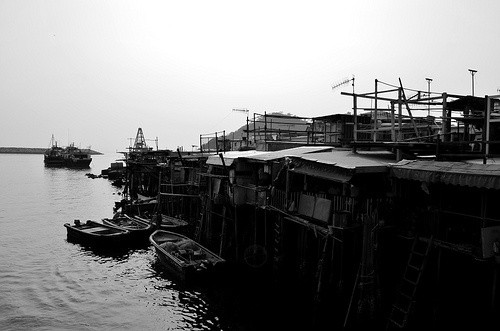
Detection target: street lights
<box><xmin>468</xmin><ymin>69</ymin><xmax>478</xmax><ymax>97</ymax></box>
<box><xmin>425</xmin><ymin>77</ymin><xmax>433</xmax><ymax>125</ymax></box>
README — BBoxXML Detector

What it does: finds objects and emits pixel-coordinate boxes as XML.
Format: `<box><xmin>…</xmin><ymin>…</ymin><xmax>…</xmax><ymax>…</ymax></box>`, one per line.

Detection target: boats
<box><xmin>65</xmin><ymin>142</ymin><xmax>92</xmax><ymax>169</ymax></box>
<box><xmin>64</xmin><ymin>209</ymin><xmax>131</xmax><ymax>252</ymax></box>
<box><xmin>149</xmin><ymin>229</ymin><xmax>227</xmax><ymax>281</ymax></box>
<box><xmin>41</xmin><ymin>134</ymin><xmax>68</xmax><ymax>167</ymax></box>
<box><xmin>101</xmin><ymin>208</ymin><xmax>189</xmax><ymax>242</ymax></box>
